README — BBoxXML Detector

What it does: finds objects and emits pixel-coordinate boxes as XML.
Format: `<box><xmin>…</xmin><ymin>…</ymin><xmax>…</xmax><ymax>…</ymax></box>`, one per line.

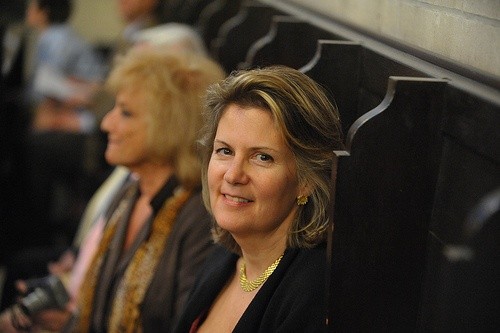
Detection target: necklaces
<box><xmin>238</xmin><ymin>251</ymin><xmax>285</xmax><ymax>292</ymax></box>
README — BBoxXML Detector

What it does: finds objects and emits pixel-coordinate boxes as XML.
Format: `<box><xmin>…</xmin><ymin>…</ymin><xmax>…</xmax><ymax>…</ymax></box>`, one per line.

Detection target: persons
<box><xmin>0</xmin><ymin>1</ymin><xmax>209</xmax><ymax>333</ymax></box>
<box><xmin>75</xmin><ymin>41</ymin><xmax>228</xmax><ymax>333</ymax></box>
<box><xmin>173</xmin><ymin>65</ymin><xmax>345</xmax><ymax>333</ymax></box>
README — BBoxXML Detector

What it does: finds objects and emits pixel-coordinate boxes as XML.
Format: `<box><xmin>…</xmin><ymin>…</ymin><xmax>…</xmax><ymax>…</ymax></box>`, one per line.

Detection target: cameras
<box><xmin>18</xmin><ymin>276</ymin><xmax>69</xmax><ymax>318</ymax></box>
